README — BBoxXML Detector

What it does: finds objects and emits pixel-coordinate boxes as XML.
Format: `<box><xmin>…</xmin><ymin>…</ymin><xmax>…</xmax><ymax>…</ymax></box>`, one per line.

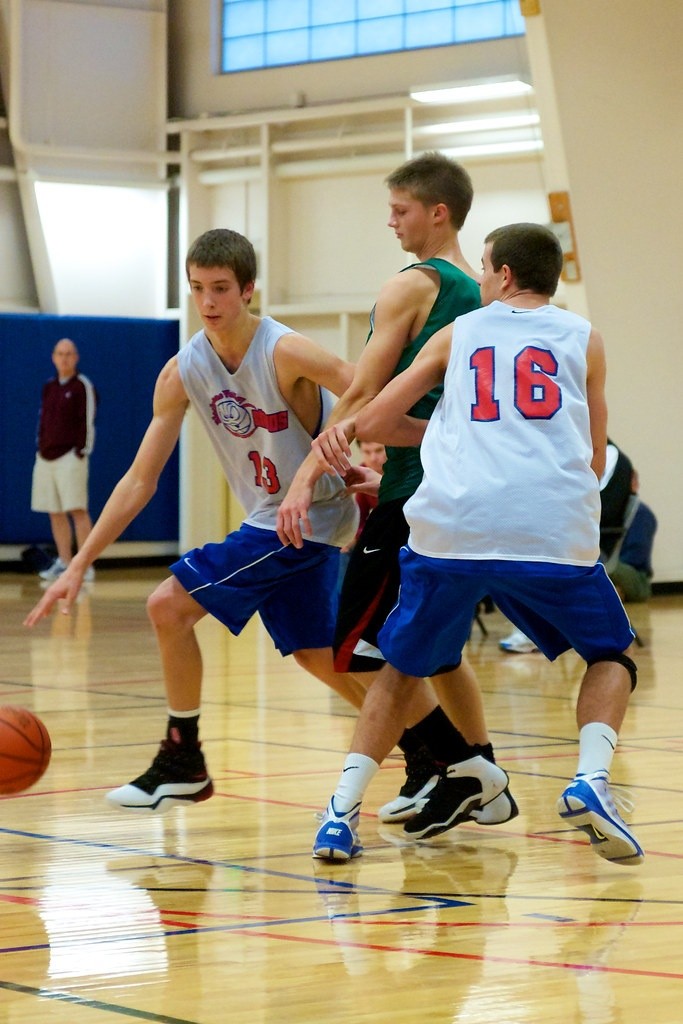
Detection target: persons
<box><xmin>339</xmin><ymin>439</ymin><xmax>388</xmax><ymax>553</ymax></box>
<box><xmin>309</xmin><ymin>223</ymin><xmax>646</xmax><ymax>866</ymax></box>
<box><xmin>29</xmin><ymin>338</ymin><xmax>98</xmax><ymax>581</ymax></box>
<box><xmin>276</xmin><ymin>153</ymin><xmax>519</xmax><ymax>841</ymax></box>
<box><xmin>498</xmin><ymin>436</ymin><xmax>632</xmax><ymax>654</ymax></box>
<box><xmin>22</xmin><ymin>229</ymin><xmax>437</xmax><ymax>822</ymax></box>
<box><xmin>607</xmin><ymin>468</ymin><xmax>657</xmax><ymax>601</ymax></box>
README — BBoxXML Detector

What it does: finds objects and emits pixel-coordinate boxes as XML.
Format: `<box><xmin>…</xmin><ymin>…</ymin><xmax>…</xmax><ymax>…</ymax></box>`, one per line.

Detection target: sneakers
<box><xmin>498</xmin><ymin>632</ymin><xmax>539</xmax><ymax>652</ymax></box>
<box><xmin>312</xmin><ymin>795</ymin><xmax>363</xmax><ymax>859</ymax></box>
<box><xmin>414</xmin><ymin>789</ymin><xmax>521</xmax><ymax>841</ymax></box>
<box><xmin>38</xmin><ymin>559</ymin><xmax>69</xmax><ymax>579</ymax></box>
<box><xmin>107</xmin><ymin>737</ymin><xmax>213</xmax><ymax>812</ymax></box>
<box><xmin>558</xmin><ymin>769</ymin><xmax>643</xmax><ymax>866</ymax></box>
<box><xmin>81</xmin><ymin>568</ymin><xmax>96</xmax><ymax>581</ymax></box>
<box><xmin>405</xmin><ymin>754</ymin><xmax>507</xmax><ymax>841</ymax></box>
<box><xmin>380</xmin><ymin>758</ymin><xmax>443</xmax><ymax>822</ymax></box>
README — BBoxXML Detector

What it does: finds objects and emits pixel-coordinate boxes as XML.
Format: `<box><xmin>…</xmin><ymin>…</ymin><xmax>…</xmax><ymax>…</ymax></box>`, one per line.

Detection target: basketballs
<box><xmin>0</xmin><ymin>703</ymin><xmax>53</xmax><ymax>798</ymax></box>
